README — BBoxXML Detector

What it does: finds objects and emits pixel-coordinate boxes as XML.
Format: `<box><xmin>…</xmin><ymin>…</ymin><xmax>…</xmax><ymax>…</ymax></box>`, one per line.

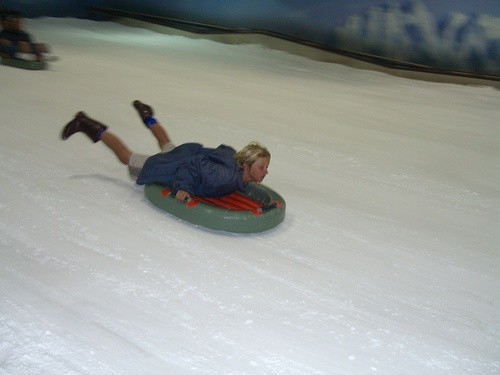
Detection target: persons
<box><xmin>0</xmin><ymin>8</ymin><xmax>59</xmax><ymax>62</ymax></box>
<box><xmin>62</xmin><ymin>100</ymin><xmax>270</xmax><ymax>203</ymax></box>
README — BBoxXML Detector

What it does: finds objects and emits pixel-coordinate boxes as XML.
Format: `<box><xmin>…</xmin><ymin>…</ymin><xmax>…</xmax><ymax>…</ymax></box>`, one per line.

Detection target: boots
<box><xmin>62</xmin><ymin>112</ymin><xmax>106</xmax><ymax>143</ymax></box>
<box><xmin>133</xmin><ymin>100</ymin><xmax>152</xmax><ymax>121</ymax></box>
